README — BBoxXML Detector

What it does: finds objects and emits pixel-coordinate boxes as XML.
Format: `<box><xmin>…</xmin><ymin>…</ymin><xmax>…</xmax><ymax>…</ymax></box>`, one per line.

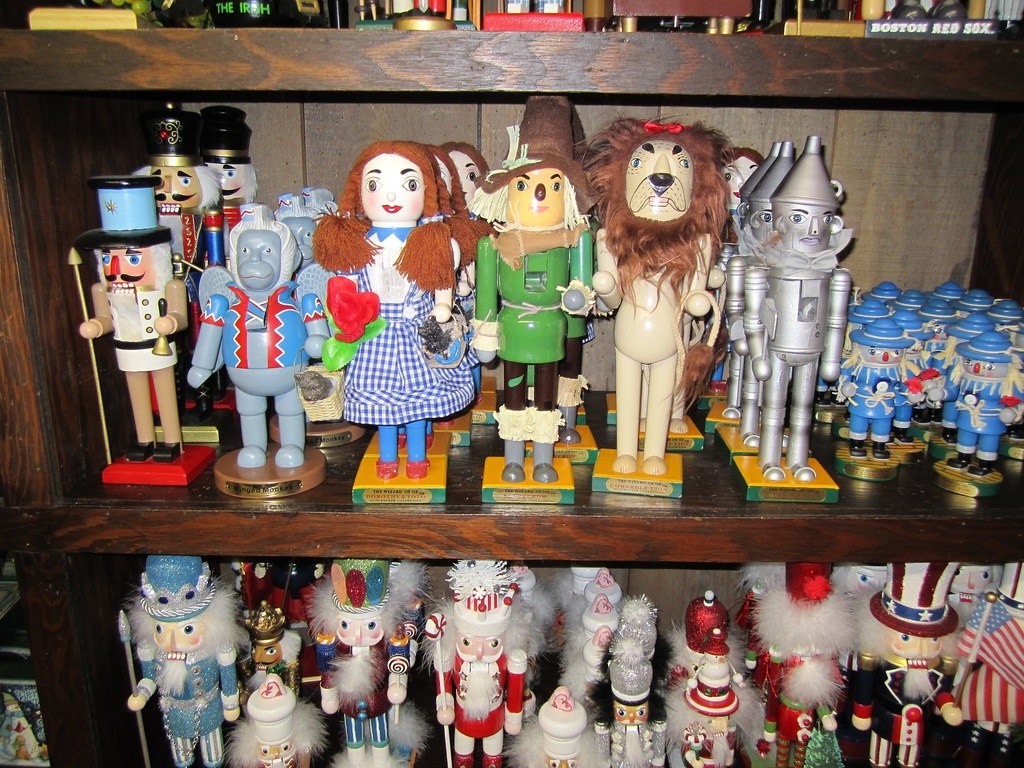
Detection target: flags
<box><xmin>958</xmin><ymin>594</ymin><xmax>1024</xmax><ymax>690</ymax></box>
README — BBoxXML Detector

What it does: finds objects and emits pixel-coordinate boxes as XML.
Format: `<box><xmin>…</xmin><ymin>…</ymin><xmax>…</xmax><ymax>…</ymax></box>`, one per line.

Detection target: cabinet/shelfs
<box><xmin>0</xmin><ymin>29</ymin><xmax>1024</xmax><ymax>768</ymax></box>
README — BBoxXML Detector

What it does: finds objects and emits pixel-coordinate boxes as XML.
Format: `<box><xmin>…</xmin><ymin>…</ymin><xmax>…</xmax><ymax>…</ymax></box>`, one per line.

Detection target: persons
<box><xmin>927</xmin><ymin>331</ymin><xmax>1024</xmax><ymax>476</ymax></box>
<box><xmin>131</xmin><ymin>107</ymin><xmax>227</xmax><ymax>424</ymax></box>
<box><xmin>312</xmin><ymin>558</ymin><xmax>427</xmax><ymax>768</ymax></box>
<box><xmin>709</xmin><ymin>140</ymin><xmax>796</xmax><ymax>450</ymax></box>
<box><xmin>592</xmin><ymin>592</ymin><xmax>672</xmax><ymax>768</ymax></box>
<box><xmin>475</xmin><ymin>95</ymin><xmax>600</xmax><ymax>485</ymax></box>
<box><xmin>734</xmin><ymin>560</ymin><xmax>836</xmax><ymax>768</ymax></box>
<box><xmin>310</xmin><ymin>139</ymin><xmax>489</xmax><ymax>477</ymax></box>
<box><xmin>194</xmin><ymin>98</ymin><xmax>259</xmax><ymax>232</ymax></box>
<box><xmin>840</xmin><ymin>318</ymin><xmax>913</xmax><ymax>461</ymax></box>
<box><xmin>745</xmin><ymin>136</ymin><xmax>851</xmax><ymax>479</ymax></box>
<box><xmin>116</xmin><ymin>554</ymin><xmax>327</xmax><ymax>768</ymax></box>
<box><xmin>68</xmin><ymin>175</ymin><xmax>187</xmax><ymax>462</ymax></box>
<box><xmin>852</xmin><ymin>563</ymin><xmax>963</xmax><ymax>768</ymax></box>
<box><xmin>426</xmin><ymin>560</ymin><xmax>528</xmax><ymax>768</ymax></box>
<box><xmin>828</xmin><ymin>564</ymin><xmax>1004</xmax><ymax>739</ymax></box>
<box><xmin>820</xmin><ymin>281</ymin><xmax>1024</xmax><ymax>444</ymax></box>
<box><xmin>504</xmin><ymin>567</ymin><xmax>623</xmax><ymax>768</ymax></box>
<box><xmin>667</xmin><ymin>592</ymin><xmax>763</xmax><ymax>768</ymax></box>
